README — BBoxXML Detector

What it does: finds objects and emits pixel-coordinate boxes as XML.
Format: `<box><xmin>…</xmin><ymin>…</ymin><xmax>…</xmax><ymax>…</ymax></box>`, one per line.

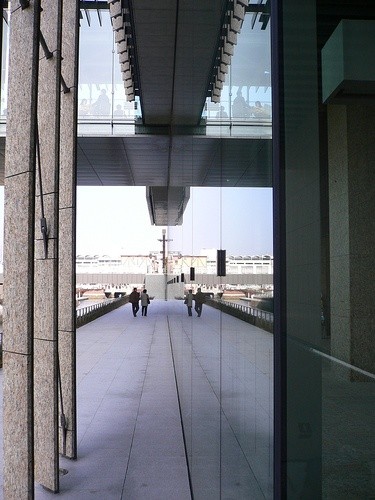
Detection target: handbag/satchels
<box><xmin>147</xmin><ymin>294</ymin><xmax>150</xmax><ymax>304</ymax></box>
<box><xmin>184</xmin><ymin>294</ymin><xmax>189</xmax><ymax>304</ymax></box>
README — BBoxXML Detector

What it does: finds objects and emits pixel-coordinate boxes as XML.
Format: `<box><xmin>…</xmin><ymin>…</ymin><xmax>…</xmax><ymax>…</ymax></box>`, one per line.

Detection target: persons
<box><xmin>186</xmin><ymin>290</ymin><xmax>194</xmax><ymax>316</ymax></box>
<box><xmin>195</xmin><ymin>288</ymin><xmax>204</xmax><ymax>317</ymax></box>
<box><xmin>141</xmin><ymin>289</ymin><xmax>149</xmax><ymax>316</ymax></box>
<box><xmin>128</xmin><ymin>288</ymin><xmax>140</xmax><ymax>317</ymax></box>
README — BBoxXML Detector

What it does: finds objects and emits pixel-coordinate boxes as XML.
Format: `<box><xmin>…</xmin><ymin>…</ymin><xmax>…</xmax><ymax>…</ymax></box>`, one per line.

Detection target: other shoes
<box><xmin>197</xmin><ymin>315</ymin><xmax>200</xmax><ymax>317</ymax></box>
<box><xmin>134</xmin><ymin>313</ymin><xmax>137</xmax><ymax>317</ymax></box>
